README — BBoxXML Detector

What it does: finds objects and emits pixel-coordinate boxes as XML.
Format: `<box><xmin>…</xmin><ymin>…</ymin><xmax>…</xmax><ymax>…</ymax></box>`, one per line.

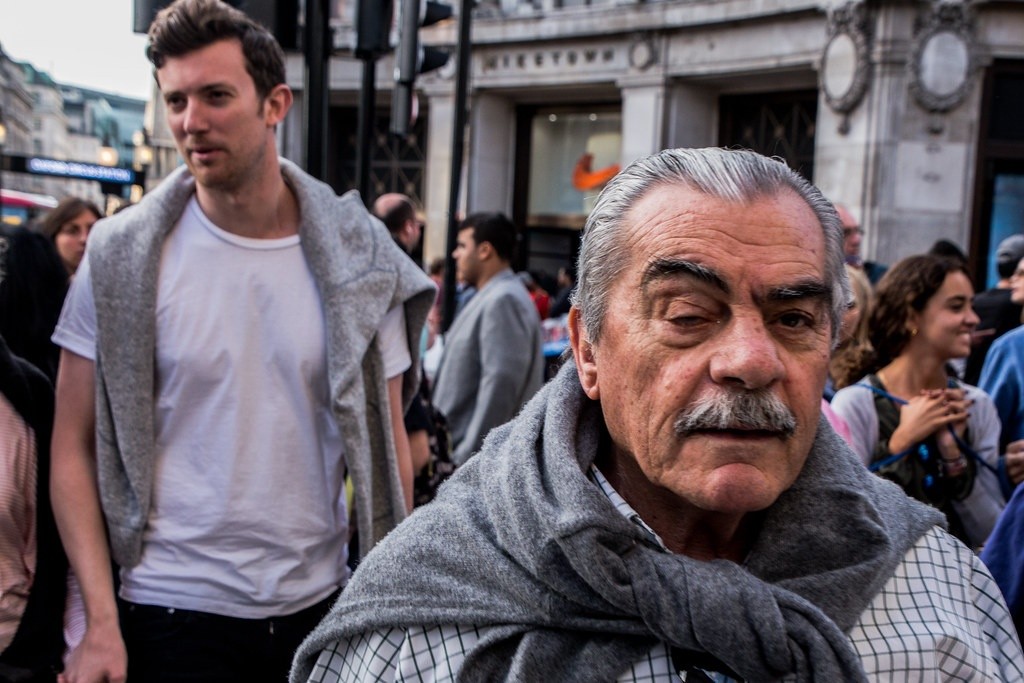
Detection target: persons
<box><xmin>53</xmin><ymin>0</ymin><xmax>435</xmax><ymax>683</ymax></box>
<box><xmin>290</xmin><ymin>148</ymin><xmax>1024</xmax><ymax>683</ymax></box>
<box><xmin>0</xmin><ymin>193</ymin><xmax>1024</xmax><ymax>683</ymax></box>
